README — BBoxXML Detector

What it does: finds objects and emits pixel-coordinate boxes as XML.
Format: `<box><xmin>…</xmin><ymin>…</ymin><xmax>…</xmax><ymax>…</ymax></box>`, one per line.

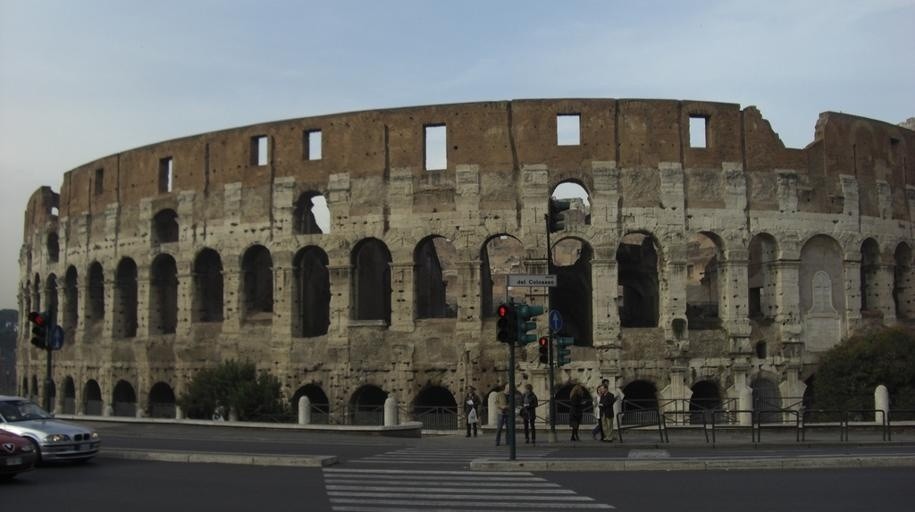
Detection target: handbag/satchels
<box><xmin>468</xmin><ymin>407</ymin><xmax>479</xmax><ymax>424</ymax></box>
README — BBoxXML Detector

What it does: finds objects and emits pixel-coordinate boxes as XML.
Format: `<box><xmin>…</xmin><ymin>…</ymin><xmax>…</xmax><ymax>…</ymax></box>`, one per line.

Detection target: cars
<box><xmin>1</xmin><ymin>396</ymin><xmax>101</xmax><ymax>481</ymax></box>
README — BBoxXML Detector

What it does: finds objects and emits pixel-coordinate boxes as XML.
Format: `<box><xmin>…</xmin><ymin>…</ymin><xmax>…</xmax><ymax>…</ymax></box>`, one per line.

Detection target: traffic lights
<box><xmin>496</xmin><ymin>304</ymin><xmax>513</xmax><ymax>342</ymax></box>
<box><xmin>29</xmin><ymin>311</ymin><xmax>49</xmax><ymax>349</ymax></box>
<box><xmin>554</xmin><ymin>332</ymin><xmax>575</xmax><ymax>368</ymax></box>
<box><xmin>539</xmin><ymin>337</ymin><xmax>548</xmax><ymax>363</ymax></box>
<box><xmin>515</xmin><ymin>305</ymin><xmax>543</xmax><ymax>346</ymax></box>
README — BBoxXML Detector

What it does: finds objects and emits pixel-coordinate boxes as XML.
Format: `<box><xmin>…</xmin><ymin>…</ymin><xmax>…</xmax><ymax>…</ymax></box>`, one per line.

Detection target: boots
<box><xmin>571</xmin><ymin>429</ymin><xmax>579</xmax><ymax>440</ymax></box>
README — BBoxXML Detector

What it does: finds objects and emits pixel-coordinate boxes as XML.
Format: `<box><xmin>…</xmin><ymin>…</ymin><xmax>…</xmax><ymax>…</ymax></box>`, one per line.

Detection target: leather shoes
<box><xmin>592</xmin><ymin>431</ymin><xmax>612</xmax><ymax>442</ymax></box>
<box><xmin>466</xmin><ymin>433</ymin><xmax>471</xmax><ymax>437</ymax></box>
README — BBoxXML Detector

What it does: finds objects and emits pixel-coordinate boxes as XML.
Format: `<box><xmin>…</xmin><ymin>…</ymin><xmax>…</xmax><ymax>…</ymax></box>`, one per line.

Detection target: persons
<box><xmin>598</xmin><ymin>385</ymin><xmax>614</xmax><ymax>444</ymax></box>
<box><xmin>495</xmin><ymin>383</ymin><xmax>515</xmax><ymax>446</ymax></box>
<box><xmin>463</xmin><ymin>386</ymin><xmax>479</xmax><ymax>438</ymax></box>
<box><xmin>567</xmin><ymin>384</ymin><xmax>587</xmax><ymax>442</ymax></box>
<box><xmin>210</xmin><ymin>400</ymin><xmax>225</xmax><ymax>422</ymax></box>
<box><xmin>513</xmin><ymin>386</ymin><xmax>522</xmax><ymax>408</ymax></box>
<box><xmin>522</xmin><ymin>382</ymin><xmax>539</xmax><ymax>444</ymax></box>
<box><xmin>602</xmin><ymin>380</ymin><xmax>610</xmax><ymax>385</ymax></box>
<box><xmin>592</xmin><ymin>386</ymin><xmax>608</xmax><ymax>439</ymax></box>
